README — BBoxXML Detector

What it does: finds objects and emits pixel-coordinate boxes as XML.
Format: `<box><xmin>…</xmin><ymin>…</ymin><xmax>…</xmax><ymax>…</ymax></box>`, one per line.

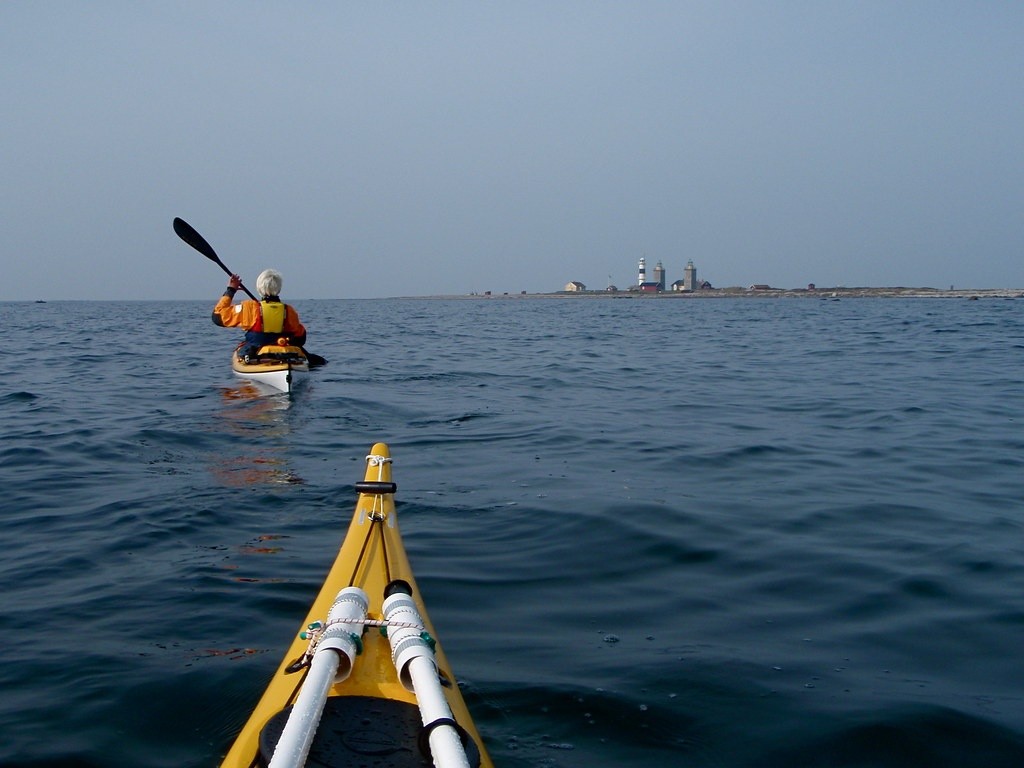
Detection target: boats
<box><xmin>218</xmin><ymin>437</ymin><xmax>494</xmax><ymax>767</ymax></box>
<box><xmin>233</xmin><ymin>345</ymin><xmax>309</xmax><ymax>397</ymax></box>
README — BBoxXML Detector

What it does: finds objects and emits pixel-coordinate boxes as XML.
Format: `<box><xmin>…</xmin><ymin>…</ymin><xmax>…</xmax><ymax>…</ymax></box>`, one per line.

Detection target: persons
<box><xmin>212</xmin><ymin>269</ymin><xmax>307</xmax><ymax>359</ymax></box>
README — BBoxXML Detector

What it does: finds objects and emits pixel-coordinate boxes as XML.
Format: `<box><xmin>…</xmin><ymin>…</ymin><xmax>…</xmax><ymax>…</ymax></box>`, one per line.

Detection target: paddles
<box><xmin>172</xmin><ymin>216</ymin><xmax>329</xmax><ymax>365</ymax></box>
